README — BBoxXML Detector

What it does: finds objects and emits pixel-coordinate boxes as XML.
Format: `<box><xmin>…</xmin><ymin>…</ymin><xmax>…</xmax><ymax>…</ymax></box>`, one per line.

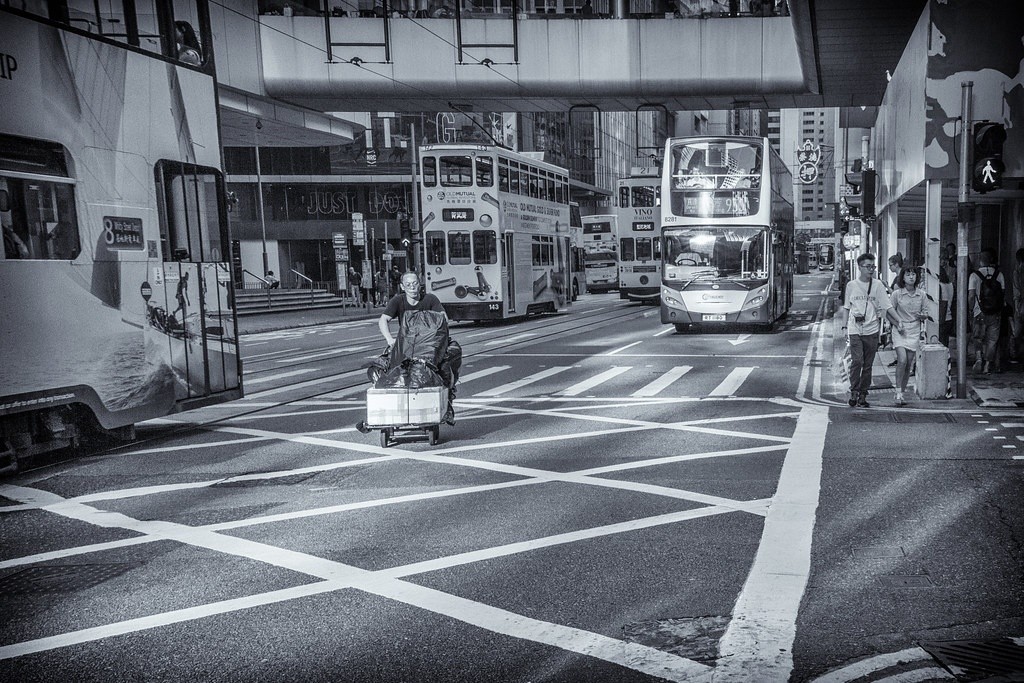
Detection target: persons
<box><xmin>886</xmin><ymin>265</ymin><xmax>928</xmax><ymax>405</ymax></box>
<box><xmin>175</xmin><ymin>20</ymin><xmax>202</xmax><ymax>65</ymax></box>
<box><xmin>968</xmin><ymin>252</ymin><xmax>1005</xmax><ymax>375</ymax></box>
<box><xmin>391</xmin><ymin>266</ymin><xmax>402</xmax><ymax>297</ymax></box>
<box><xmin>349</xmin><ymin>267</ymin><xmax>360</xmax><ymax>307</ymax></box>
<box><xmin>264</xmin><ymin>271</ymin><xmax>279</xmax><ymax>289</ymax></box>
<box><xmin>173</xmin><ymin>272</ymin><xmax>190</xmax><ymax>320</ymax></box>
<box><xmin>379</xmin><ymin>271</ymin><xmax>448</xmax><ymax>347</ymax></box>
<box><xmin>882</xmin><ymin>243</ymin><xmax>956</xmax><ymax>366</ymax></box>
<box><xmin>376</xmin><ymin>268</ymin><xmax>386</xmax><ymax>307</ymax></box>
<box><xmin>841</xmin><ymin>254</ymin><xmax>904</xmax><ymax>407</ymax></box>
<box><xmin>1010</xmin><ymin>248</ymin><xmax>1024</xmax><ymax>363</ymax></box>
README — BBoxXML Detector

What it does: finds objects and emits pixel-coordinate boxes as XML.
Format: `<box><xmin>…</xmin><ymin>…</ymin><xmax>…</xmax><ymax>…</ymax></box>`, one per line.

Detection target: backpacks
<box><xmin>974</xmin><ymin>270</ymin><xmax>1003</xmax><ymax>315</ymax></box>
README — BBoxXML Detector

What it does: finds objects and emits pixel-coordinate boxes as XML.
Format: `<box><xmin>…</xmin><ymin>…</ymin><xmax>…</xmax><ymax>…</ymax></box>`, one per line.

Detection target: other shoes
<box><xmin>858</xmin><ymin>398</ymin><xmax>869</xmax><ymax>407</ymax></box>
<box><xmin>848</xmin><ymin>391</ymin><xmax>858</xmax><ymax>406</ymax></box>
<box><xmin>894</xmin><ymin>392</ymin><xmax>907</xmax><ymax>405</ymax></box>
<box><xmin>972</xmin><ymin>360</ymin><xmax>982</xmax><ymax>372</ymax></box>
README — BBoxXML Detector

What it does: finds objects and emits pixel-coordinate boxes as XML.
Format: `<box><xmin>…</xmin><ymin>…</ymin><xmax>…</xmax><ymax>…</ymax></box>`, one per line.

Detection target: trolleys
<box><xmin>365</xmin><ymin>345</ymin><xmax>450</xmax><ymax>448</ymax></box>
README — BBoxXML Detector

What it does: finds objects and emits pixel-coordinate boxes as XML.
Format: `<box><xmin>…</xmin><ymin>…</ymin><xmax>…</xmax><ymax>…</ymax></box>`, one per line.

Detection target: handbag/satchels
<box><xmin>838</xmin><ymin>342</ymin><xmax>853</xmax><ymax>381</ymax></box>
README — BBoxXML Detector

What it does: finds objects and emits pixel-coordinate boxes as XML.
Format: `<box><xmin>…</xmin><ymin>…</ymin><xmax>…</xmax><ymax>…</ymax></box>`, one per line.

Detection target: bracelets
<box><xmin>842</xmin><ymin>327</ymin><xmax>847</xmax><ymax>330</ymax></box>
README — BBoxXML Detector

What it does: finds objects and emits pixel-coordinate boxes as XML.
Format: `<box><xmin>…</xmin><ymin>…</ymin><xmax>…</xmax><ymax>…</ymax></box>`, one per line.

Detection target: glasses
<box><xmin>862</xmin><ymin>266</ymin><xmax>877</xmax><ymax>271</ymax></box>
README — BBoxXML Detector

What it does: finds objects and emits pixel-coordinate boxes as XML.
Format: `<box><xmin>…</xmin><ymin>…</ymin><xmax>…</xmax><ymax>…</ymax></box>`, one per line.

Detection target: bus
<box><xmin>653</xmin><ymin>135</ymin><xmax>796</xmax><ymax>333</ymax></box>
<box><xmin>569</xmin><ymin>167</ymin><xmax>662</xmax><ymax>306</ymax></box>
<box><xmin>0</xmin><ymin>4</ymin><xmax>247</xmax><ymax>479</ymax></box>
<box><xmin>418</xmin><ymin>101</ymin><xmax>571</xmax><ymax>326</ymax></box>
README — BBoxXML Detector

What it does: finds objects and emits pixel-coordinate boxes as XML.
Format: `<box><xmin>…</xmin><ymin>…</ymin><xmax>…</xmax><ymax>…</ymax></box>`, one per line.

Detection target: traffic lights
<box><xmin>972</xmin><ymin>121</ymin><xmax>1006</xmax><ymax>196</ymax></box>
<box><xmin>844</xmin><ymin>168</ymin><xmax>876</xmax><ymax>216</ymax></box>
<box><xmin>399</xmin><ymin>218</ymin><xmax>411</xmax><ymax>247</ymax></box>
